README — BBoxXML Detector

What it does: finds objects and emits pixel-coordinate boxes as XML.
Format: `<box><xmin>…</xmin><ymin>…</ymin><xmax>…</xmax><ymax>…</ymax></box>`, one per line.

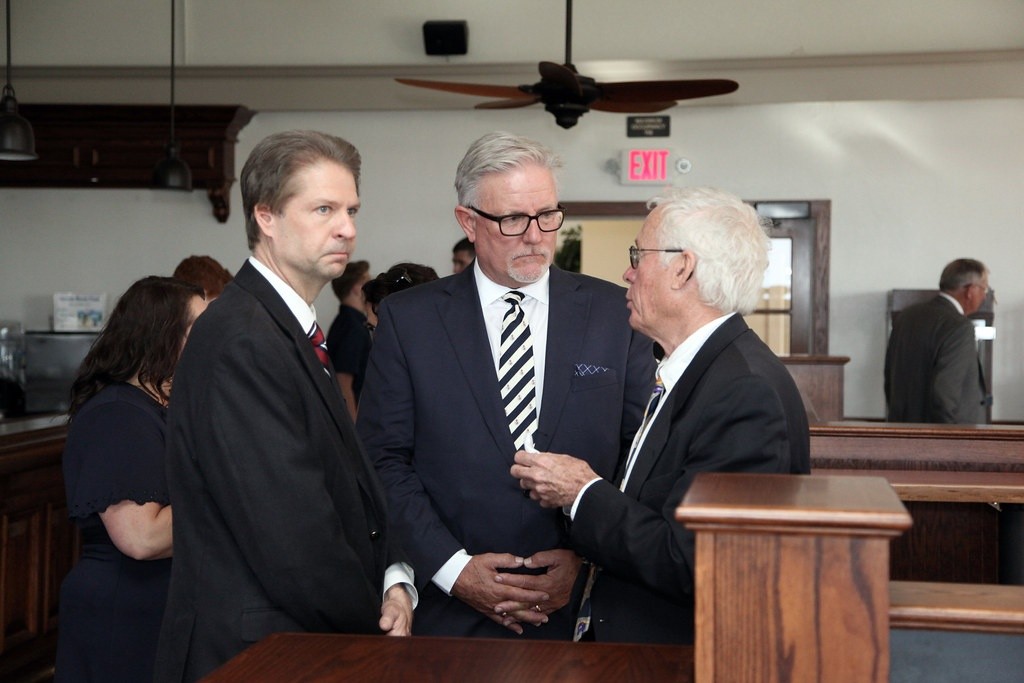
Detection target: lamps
<box><xmin>141</xmin><ymin>0</ymin><xmax>192</xmax><ymax>192</ymax></box>
<box><xmin>0</xmin><ymin>0</ymin><xmax>39</xmax><ymax>161</ymax></box>
<box><xmin>607</xmin><ymin>149</ymin><xmax>692</xmax><ymax>187</ymax></box>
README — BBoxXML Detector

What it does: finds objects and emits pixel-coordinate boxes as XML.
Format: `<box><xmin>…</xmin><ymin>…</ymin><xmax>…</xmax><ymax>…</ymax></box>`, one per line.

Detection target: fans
<box><xmin>394</xmin><ymin>0</ymin><xmax>739</xmax><ymax>130</ymax></box>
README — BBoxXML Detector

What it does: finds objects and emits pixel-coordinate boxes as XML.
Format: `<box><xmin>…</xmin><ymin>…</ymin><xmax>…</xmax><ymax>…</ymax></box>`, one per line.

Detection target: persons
<box><xmin>453</xmin><ymin>237</ymin><xmax>476</xmax><ymax>274</ymax></box>
<box><xmin>358</xmin><ymin>132</ymin><xmax>655</xmax><ymax>640</ymax></box>
<box><xmin>173</xmin><ymin>255</ymin><xmax>233</xmax><ymax>302</ymax></box>
<box><xmin>884</xmin><ymin>259</ymin><xmax>989</xmax><ymax>423</ymax></box>
<box><xmin>362</xmin><ymin>263</ymin><xmax>439</xmax><ymax>316</ymax></box>
<box><xmin>326</xmin><ymin>261</ymin><xmax>371</xmax><ymax>424</ymax></box>
<box><xmin>510</xmin><ymin>185</ymin><xmax>809</xmax><ymax>642</ymax></box>
<box><xmin>54</xmin><ymin>275</ymin><xmax>208</xmax><ymax>683</ymax></box>
<box><xmin>155</xmin><ymin>132</ymin><xmax>420</xmax><ymax>683</ymax></box>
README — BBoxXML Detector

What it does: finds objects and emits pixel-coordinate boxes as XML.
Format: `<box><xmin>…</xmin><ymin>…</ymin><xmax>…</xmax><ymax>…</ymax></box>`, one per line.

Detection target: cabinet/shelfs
<box><xmin>0</xmin><ymin>425</ymin><xmax>104</xmax><ymax>651</ymax></box>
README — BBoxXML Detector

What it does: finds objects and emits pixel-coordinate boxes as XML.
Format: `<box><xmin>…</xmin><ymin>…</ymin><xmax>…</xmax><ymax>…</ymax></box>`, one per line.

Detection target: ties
<box><xmin>308</xmin><ymin>321</ymin><xmax>332</xmax><ymax>384</ymax></box>
<box><xmin>498</xmin><ymin>290</ymin><xmax>538</xmax><ymax>451</ymax></box>
<box><xmin>572</xmin><ymin>364</ymin><xmax>667</xmax><ymax>642</ymax></box>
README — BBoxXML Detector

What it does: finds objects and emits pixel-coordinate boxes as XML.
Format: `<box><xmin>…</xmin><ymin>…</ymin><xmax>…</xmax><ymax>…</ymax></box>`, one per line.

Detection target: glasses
<box><xmin>962</xmin><ymin>283</ymin><xmax>990</xmax><ymax>295</ymax></box>
<box><xmin>629</xmin><ymin>245</ymin><xmax>684</xmax><ymax>269</ymax></box>
<box><xmin>384</xmin><ymin>267</ymin><xmax>415</xmax><ymax>286</ymax></box>
<box><xmin>468</xmin><ymin>205</ymin><xmax>568</xmax><ymax>236</ymax></box>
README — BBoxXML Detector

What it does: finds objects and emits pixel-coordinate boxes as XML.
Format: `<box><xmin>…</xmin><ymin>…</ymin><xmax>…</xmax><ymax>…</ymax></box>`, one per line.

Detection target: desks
<box><xmin>190</xmin><ymin>633</ymin><xmax>694</xmax><ymax>683</ymax></box>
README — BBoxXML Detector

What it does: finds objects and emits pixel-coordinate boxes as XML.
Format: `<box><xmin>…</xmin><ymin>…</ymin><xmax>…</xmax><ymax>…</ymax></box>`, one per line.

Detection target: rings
<box><xmin>535</xmin><ymin>605</ymin><xmax>541</xmax><ymax>611</ymax></box>
